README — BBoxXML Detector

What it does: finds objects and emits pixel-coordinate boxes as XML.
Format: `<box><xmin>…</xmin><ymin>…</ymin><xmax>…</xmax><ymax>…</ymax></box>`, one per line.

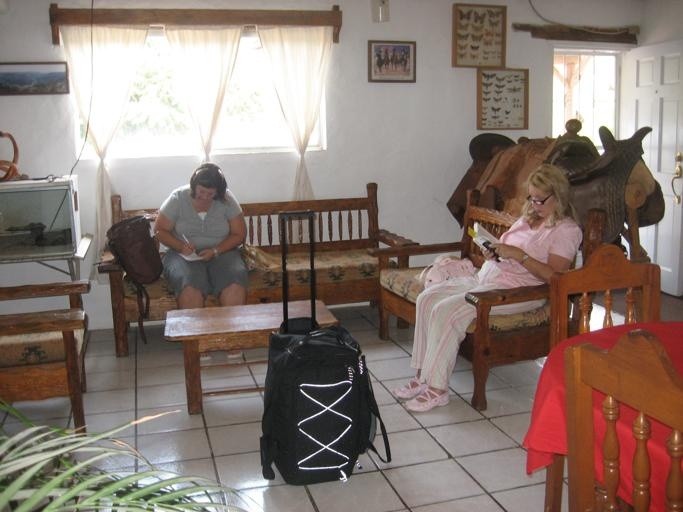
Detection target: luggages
<box><xmin>261</xmin><ymin>211</ymin><xmax>390</xmax><ymax>485</ymax></box>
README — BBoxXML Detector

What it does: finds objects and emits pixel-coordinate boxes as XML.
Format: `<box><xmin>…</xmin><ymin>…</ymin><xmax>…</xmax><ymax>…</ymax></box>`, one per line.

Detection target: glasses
<box><xmin>526</xmin><ymin>194</ymin><xmax>553</xmax><ymax>205</ymax></box>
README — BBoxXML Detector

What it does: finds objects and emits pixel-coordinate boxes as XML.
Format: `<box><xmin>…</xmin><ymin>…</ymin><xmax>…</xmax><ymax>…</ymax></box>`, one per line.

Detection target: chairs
<box><xmin>566</xmin><ymin>328</ymin><xmax>681</xmax><ymax>512</ymax></box>
<box><xmin>1</xmin><ymin>278</ymin><xmax>91</xmax><ymax>427</ymax></box>
<box><xmin>543</xmin><ymin>243</ymin><xmax>661</xmax><ymax>357</ymax></box>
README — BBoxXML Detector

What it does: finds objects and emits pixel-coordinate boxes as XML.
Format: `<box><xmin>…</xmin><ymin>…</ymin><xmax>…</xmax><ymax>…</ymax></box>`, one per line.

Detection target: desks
<box><xmin>161</xmin><ymin>298</ymin><xmax>338</xmax><ymax>414</ymax></box>
<box><xmin>535</xmin><ymin>320</ymin><xmax>683</xmax><ymax>510</ymax></box>
<box><xmin>1</xmin><ymin>234</ymin><xmax>96</xmax><ymax>287</ymax></box>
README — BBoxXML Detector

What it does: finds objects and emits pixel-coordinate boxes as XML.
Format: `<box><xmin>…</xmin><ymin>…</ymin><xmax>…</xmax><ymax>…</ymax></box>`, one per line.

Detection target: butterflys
<box><xmin>481</xmin><ymin>68</ymin><xmax>524</xmax><ymax>128</ymax></box>
<box><xmin>459</xmin><ymin>4</ymin><xmax>503</xmax><ymax>63</ymax></box>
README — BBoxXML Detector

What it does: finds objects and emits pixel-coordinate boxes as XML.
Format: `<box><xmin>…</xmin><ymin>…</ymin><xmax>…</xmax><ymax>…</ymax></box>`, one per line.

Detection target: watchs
<box><xmin>211</xmin><ymin>246</ymin><xmax>218</xmax><ymax>257</ymax></box>
<box><xmin>519</xmin><ymin>253</ymin><xmax>529</xmax><ymax>265</ymax></box>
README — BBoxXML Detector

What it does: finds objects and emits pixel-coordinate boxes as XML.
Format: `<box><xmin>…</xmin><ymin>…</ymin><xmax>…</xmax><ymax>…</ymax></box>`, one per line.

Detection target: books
<box><xmin>471</xmin><ymin>222</ymin><xmax>505</xmax><ymax>263</ymax></box>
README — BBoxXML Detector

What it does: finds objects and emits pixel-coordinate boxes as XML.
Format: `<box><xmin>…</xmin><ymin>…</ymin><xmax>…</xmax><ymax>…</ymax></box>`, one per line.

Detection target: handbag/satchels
<box><xmin>106</xmin><ymin>216</ymin><xmax>163</xmax><ymax>284</ymax></box>
<box><xmin>424</xmin><ymin>256</ymin><xmax>474</xmax><ymax>289</ymax></box>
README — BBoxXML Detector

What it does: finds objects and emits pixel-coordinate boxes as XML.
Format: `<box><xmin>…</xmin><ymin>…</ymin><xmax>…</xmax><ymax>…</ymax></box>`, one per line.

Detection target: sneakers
<box><xmin>407</xmin><ymin>388</ymin><xmax>450</xmax><ymax>412</ymax></box>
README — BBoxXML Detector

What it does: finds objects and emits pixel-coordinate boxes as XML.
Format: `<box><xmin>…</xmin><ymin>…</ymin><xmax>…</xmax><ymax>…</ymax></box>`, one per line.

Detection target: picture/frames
<box><xmin>477</xmin><ymin>67</ymin><xmax>529</xmax><ymax>131</ymax></box>
<box><xmin>1</xmin><ymin>60</ymin><xmax>69</xmax><ymax>95</ymax></box>
<box><xmin>367</xmin><ymin>39</ymin><xmax>416</xmax><ymax>83</ymax></box>
<box><xmin>452</xmin><ymin>4</ymin><xmax>506</xmax><ymax>69</ymax></box>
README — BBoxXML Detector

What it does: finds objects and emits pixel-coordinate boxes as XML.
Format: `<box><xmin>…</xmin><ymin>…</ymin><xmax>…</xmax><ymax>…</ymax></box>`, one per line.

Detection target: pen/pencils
<box><xmin>182</xmin><ymin>234</ymin><xmax>192</xmax><ymax>250</ymax></box>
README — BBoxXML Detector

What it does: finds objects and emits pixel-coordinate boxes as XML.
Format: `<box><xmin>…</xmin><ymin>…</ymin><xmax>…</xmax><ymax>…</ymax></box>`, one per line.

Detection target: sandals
<box><xmin>395</xmin><ymin>376</ymin><xmax>428</xmax><ymax>398</ymax></box>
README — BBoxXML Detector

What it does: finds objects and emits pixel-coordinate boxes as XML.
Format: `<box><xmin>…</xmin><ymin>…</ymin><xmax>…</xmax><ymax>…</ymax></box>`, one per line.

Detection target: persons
<box><xmin>375</xmin><ymin>46</ymin><xmax>409</xmax><ymax>75</ymax></box>
<box><xmin>394</xmin><ymin>164</ymin><xmax>584</xmax><ymax>411</ymax></box>
<box><xmin>153</xmin><ymin>162</ymin><xmax>248</xmax><ymax>362</ymax></box>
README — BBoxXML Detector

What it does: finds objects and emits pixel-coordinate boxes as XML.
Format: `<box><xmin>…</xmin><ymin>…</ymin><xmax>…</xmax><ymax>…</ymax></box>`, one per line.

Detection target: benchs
<box><xmin>378</xmin><ymin>191</ymin><xmax>604</xmax><ymax>411</ymax></box>
<box><xmin>100</xmin><ymin>183</ymin><xmax>419</xmax><ymax>359</ymax></box>
